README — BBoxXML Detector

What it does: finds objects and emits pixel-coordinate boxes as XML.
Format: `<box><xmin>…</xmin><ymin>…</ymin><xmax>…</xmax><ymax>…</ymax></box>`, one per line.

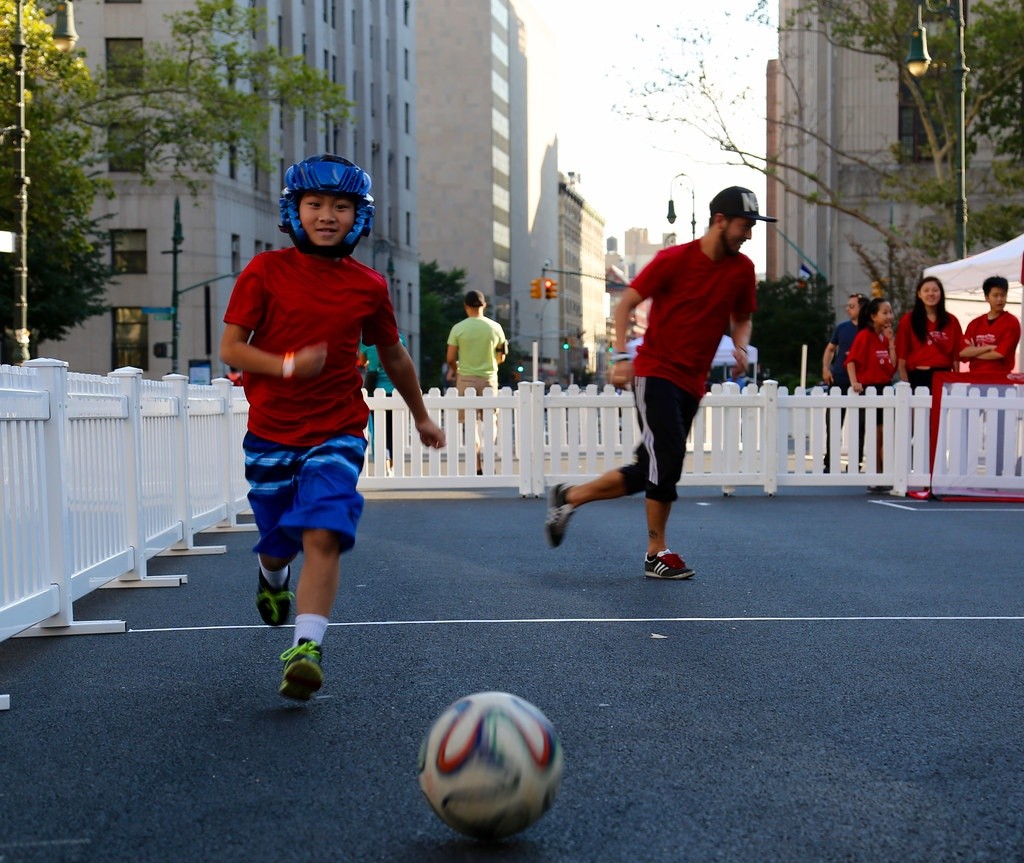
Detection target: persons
<box><xmin>546</xmin><ymin>185</ymin><xmax>777</xmax><ymax>578</ymax></box>
<box><xmin>959</xmin><ymin>276</ymin><xmax>1021</xmax><ymax>372</ymax></box>
<box><xmin>446</xmin><ymin>291</ymin><xmax>508</xmax><ymax>475</ymax></box>
<box><xmin>896</xmin><ymin>277</ymin><xmax>962</xmax><ymax>492</ymax></box>
<box><xmin>846</xmin><ymin>298</ymin><xmax>896</xmax><ymax>494</ymax></box>
<box><xmin>221</xmin><ymin>154</ymin><xmax>447</xmax><ymax>700</ymax></box>
<box><xmin>823</xmin><ymin>292</ymin><xmax>870</xmax><ymax>472</ymax></box>
<box><xmin>358</xmin><ymin>333</ymin><xmax>407</xmax><ymax>475</ymax></box>
<box><xmin>225</xmin><ymin>366</ymin><xmax>244</xmax><ymax>386</ymax></box>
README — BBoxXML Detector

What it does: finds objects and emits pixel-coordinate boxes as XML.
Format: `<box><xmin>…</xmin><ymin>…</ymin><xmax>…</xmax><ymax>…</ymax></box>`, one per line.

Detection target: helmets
<box><xmin>278</xmin><ymin>154</ymin><xmax>375</xmax><ymax>257</ymax></box>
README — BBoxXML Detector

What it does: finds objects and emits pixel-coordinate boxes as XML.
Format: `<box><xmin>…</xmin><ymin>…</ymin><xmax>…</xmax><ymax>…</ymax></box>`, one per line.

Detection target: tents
<box><xmin>923</xmin><ymin>234</ymin><xmax>1024</xmax><ymax>375</ymax></box>
<box><xmin>628</xmin><ymin>335</ymin><xmax>757</xmax><ymax>384</ymax></box>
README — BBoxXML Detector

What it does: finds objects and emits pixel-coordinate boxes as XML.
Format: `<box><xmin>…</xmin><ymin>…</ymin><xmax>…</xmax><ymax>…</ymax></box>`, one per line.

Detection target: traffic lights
<box><xmin>562</xmin><ymin>342</ymin><xmax>570</xmax><ymax>350</ymax></box>
<box><xmin>545</xmin><ymin>277</ymin><xmax>558</xmax><ymax>299</ymax></box>
<box><xmin>608</xmin><ymin>346</ymin><xmax>614</xmax><ymax>353</ymax></box>
<box><xmin>516</xmin><ymin>365</ymin><xmax>524</xmax><ymax>373</ymax></box>
<box><xmin>609</xmin><ymin>359</ymin><xmax>614</xmax><ymax>367</ymax></box>
<box><xmin>871</xmin><ymin>281</ymin><xmax>882</xmax><ymax>298</ymax></box>
<box><xmin>530</xmin><ymin>279</ymin><xmax>542</xmax><ymax>299</ymax></box>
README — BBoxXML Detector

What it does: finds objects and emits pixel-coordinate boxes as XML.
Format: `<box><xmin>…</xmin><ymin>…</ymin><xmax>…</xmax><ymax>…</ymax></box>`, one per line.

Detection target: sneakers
<box><xmin>645</xmin><ymin>549</ymin><xmax>695</xmax><ymax>580</ymax></box>
<box><xmin>545</xmin><ymin>483</ymin><xmax>575</xmax><ymax>549</ymax></box>
<box><xmin>254</xmin><ymin>565</ymin><xmax>292</xmax><ymax>628</ymax></box>
<box><xmin>277</xmin><ymin>636</ymin><xmax>325</xmax><ymax>704</ymax></box>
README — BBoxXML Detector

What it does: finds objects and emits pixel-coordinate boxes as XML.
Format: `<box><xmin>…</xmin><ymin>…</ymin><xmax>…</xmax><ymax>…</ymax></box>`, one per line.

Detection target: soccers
<box><xmin>417</xmin><ymin>691</ymin><xmax>564</xmax><ymax>839</ymax></box>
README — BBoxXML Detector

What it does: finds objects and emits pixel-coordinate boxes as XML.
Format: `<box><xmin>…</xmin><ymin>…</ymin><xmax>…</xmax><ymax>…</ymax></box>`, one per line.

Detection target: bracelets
<box><xmin>283</xmin><ymin>352</ymin><xmax>295</xmax><ymax>378</ymax></box>
<box><xmin>740</xmin><ymin>347</ymin><xmax>748</xmax><ymax>354</ymax></box>
<box><xmin>614</xmin><ymin>352</ymin><xmax>632</xmax><ymax>361</ymax></box>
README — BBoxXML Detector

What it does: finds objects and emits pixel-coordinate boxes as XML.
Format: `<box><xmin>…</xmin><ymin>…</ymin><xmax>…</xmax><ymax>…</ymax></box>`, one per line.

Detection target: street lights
<box><xmin>160</xmin><ymin>193</ymin><xmax>247</xmax><ymax>374</ymax></box>
<box><xmin>666</xmin><ymin>173</ymin><xmax>697</xmax><ymax>241</ymax></box>
<box><xmin>10</xmin><ymin>0</ymin><xmax>78</xmax><ymax>364</ymax></box>
<box><xmin>905</xmin><ymin>0</ymin><xmax>965</xmax><ymax>262</ymax></box>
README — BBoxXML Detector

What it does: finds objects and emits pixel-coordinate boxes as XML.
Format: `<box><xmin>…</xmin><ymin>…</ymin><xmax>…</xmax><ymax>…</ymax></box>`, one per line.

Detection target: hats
<box><xmin>709</xmin><ymin>187</ymin><xmax>778</xmax><ymax>223</ymax></box>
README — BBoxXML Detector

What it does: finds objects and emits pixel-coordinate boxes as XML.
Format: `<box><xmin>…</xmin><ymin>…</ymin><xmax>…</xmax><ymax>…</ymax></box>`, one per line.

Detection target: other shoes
<box><xmin>866</xmin><ymin>485</ymin><xmax>893</xmax><ymax>493</ymax></box>
